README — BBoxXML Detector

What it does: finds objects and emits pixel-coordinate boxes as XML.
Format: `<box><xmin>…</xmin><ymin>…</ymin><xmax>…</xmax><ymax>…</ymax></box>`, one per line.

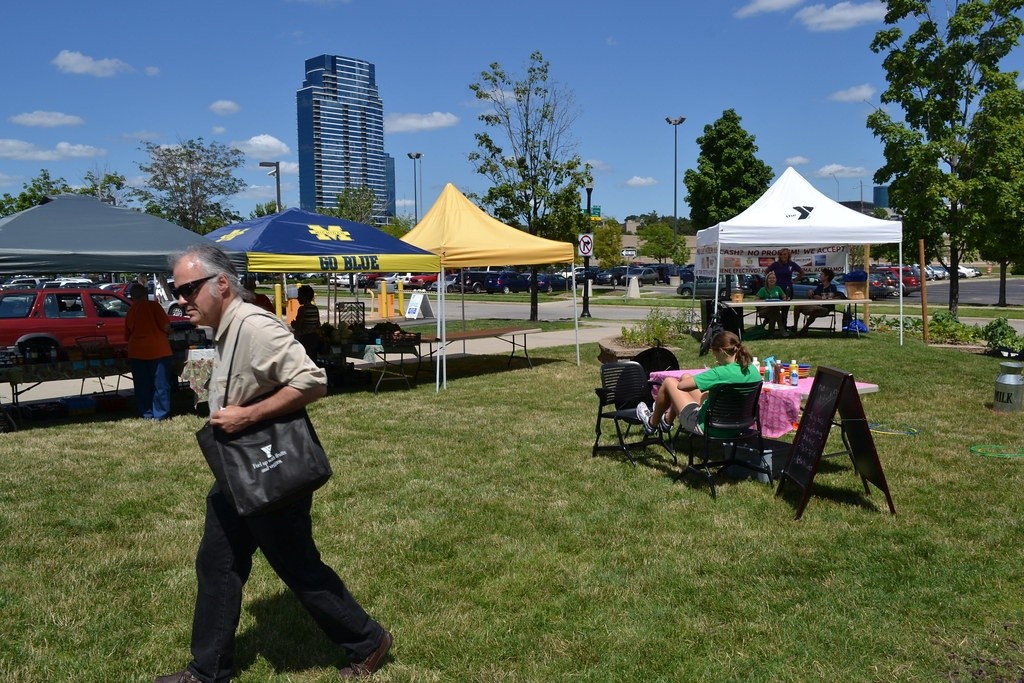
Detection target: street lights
<box><xmin>259</xmin><ymin>161</ymin><xmax>281</xmax><ymax>213</ymax></box>
<box><xmin>407</xmin><ymin>151</ymin><xmax>422</xmax><ymax>227</ymax></box>
<box><xmin>580</xmin><ymin>179</ymin><xmax>594</xmax><ymax>317</ymax></box>
<box><xmin>828</xmin><ymin>172</ymin><xmax>839</xmax><ymax>202</ymax></box>
<box><xmin>665</xmin><ymin>115</ymin><xmax>686</xmax><ymax>245</ymax></box>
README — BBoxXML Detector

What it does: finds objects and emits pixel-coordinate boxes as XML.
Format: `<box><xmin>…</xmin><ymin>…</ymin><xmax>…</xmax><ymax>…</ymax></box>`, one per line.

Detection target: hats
<box><xmin>129</xmin><ymin>284</ymin><xmax>146</xmax><ymax>300</ymax></box>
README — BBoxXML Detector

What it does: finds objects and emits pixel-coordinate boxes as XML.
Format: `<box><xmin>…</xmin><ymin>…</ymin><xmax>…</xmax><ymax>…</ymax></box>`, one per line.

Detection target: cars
<box><xmin>791</xmin><ymin>263</ymin><xmax>982</xmax><ymax>300</ymax></box>
<box><xmin>287</xmin><ymin>265</ymin><xmax>578</xmax><ymax>294</ymax></box>
<box><xmin>0</xmin><ymin>273</ymin><xmax>186</xmax><ymax>363</ymax></box>
<box><xmin>555</xmin><ymin>262</ymin><xmax>765</xmax><ymax>298</ymax></box>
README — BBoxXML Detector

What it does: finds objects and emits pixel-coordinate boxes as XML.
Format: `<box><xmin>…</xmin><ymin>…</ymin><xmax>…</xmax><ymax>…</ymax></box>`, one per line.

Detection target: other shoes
<box><xmin>798</xmin><ymin>328</ymin><xmax>808</xmax><ymax>335</ymax></box>
<box><xmin>787</xmin><ymin>326</ymin><xmax>797</xmax><ymax>332</ymax></box>
<box><xmin>767</xmin><ymin>330</ymin><xmax>778</xmax><ymax>338</ymax></box>
<box><xmin>636</xmin><ymin>402</ymin><xmax>657</xmax><ymax>436</ymax></box>
<box><xmin>652</xmin><ymin>401</ymin><xmax>672</xmax><ymax>433</ymax></box>
<box><xmin>779</xmin><ymin>327</ymin><xmax>790</xmax><ymax>337</ymax></box>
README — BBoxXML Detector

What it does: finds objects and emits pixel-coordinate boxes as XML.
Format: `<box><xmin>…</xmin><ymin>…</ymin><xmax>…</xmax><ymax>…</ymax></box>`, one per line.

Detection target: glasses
<box><xmin>172</xmin><ymin>272</ymin><xmax>215</xmax><ymax>300</ymax></box>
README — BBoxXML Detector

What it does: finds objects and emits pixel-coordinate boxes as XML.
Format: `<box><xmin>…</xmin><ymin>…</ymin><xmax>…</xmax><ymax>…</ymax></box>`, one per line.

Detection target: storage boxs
<box><xmin>845</xmin><ymin>282</ymin><xmax>867</xmax><ymax>300</ymax></box>
<box><xmin>723</xmin><ymin>437</ymin><xmax>793</xmax><ymax>483</ymax></box>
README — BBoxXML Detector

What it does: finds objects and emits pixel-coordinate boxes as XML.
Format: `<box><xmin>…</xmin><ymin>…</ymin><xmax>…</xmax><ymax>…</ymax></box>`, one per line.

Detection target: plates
<box><xmin>781</xmin><ymin>363</ymin><xmax>811</xmax><ymax>378</ymax></box>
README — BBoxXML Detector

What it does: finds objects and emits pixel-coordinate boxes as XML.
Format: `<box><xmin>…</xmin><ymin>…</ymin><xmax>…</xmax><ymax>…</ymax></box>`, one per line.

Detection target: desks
<box><xmin>650</xmin><ymin>369</ymin><xmax>878</xmax><ymax>478</ymax></box>
<box><xmin>420</xmin><ymin>327</ymin><xmax>541</xmax><ymax>374</ymax></box>
<box><xmin>723</xmin><ymin>299</ymin><xmax>877</xmax><ymax>340</ymax></box>
<box><xmin>323</xmin><ymin>338</ymin><xmax>419</xmax><ymax>393</ymax></box>
<box><xmin>0</xmin><ymin>346</ymin><xmax>134</xmax><ymax>405</ymax></box>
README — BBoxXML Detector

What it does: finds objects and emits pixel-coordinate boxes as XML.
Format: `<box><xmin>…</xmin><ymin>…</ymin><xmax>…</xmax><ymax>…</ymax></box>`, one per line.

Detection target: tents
<box><xmin>399</xmin><ymin>183</ymin><xmax>581</xmax><ymax>391</ymax></box>
<box><xmin>202</xmin><ymin>207</ymin><xmax>441</xmax><ymax>393</ymax></box>
<box><xmin>123</xmin><ymin>284</ymin><xmax>175</xmax><ymax>422</ymax></box>
<box><xmin>690</xmin><ymin>166</ymin><xmax>902</xmax><ymax>346</ymax></box>
<box><xmin>0</xmin><ymin>193</ymin><xmax>248</xmax><ymax>277</ymax></box>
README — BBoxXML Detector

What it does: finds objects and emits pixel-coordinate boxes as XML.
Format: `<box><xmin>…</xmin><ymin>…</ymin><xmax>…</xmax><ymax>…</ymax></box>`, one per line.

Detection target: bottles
<box><xmin>0</xmin><ymin>353</ymin><xmax>24</xmax><ymax>369</ymax></box>
<box><xmin>827</xmin><ymin>286</ymin><xmax>833</xmax><ymax>300</ymax></box>
<box><xmin>821</xmin><ymin>286</ymin><xmax>826</xmax><ymax>299</ymax></box>
<box><xmin>31</xmin><ymin>347</ymin><xmax>38</xmax><ymax>364</ymax></box>
<box><xmin>50</xmin><ymin>345</ymin><xmax>57</xmax><ymax>362</ymax></box>
<box><xmin>25</xmin><ymin>347</ymin><xmax>32</xmax><ymax>365</ymax></box>
<box><xmin>763</xmin><ymin>359</ymin><xmax>798</xmax><ymax>386</ymax></box>
<box><xmin>785</xmin><ymin>286</ymin><xmax>792</xmax><ymax>301</ymax></box>
<box><xmin>752</xmin><ymin>357</ymin><xmax>760</xmax><ymax>372</ymax></box>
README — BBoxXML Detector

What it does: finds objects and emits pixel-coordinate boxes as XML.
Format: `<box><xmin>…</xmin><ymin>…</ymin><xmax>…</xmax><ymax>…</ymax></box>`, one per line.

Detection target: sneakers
<box><xmin>156</xmin><ymin>668</ymin><xmax>204</xmax><ymax>683</ymax></box>
<box><xmin>339</xmin><ymin>631</ymin><xmax>393</xmax><ymax>679</ymax></box>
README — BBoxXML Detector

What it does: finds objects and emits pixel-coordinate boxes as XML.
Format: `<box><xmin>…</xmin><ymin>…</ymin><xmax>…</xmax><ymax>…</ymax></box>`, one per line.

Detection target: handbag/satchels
<box><xmin>844</xmin><ymin>318</ymin><xmax>867</xmax><ymax>333</ymax></box>
<box><xmin>194</xmin><ymin>313</ymin><xmax>332</xmax><ymax>519</ymax></box>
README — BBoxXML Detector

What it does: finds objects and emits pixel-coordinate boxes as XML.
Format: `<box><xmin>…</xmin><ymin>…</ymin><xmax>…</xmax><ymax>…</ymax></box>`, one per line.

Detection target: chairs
<box><xmin>592</xmin><ymin>361</ymin><xmax>677</xmax><ymax>468</ymax></box>
<box><xmin>672</xmin><ymin>378</ymin><xmax>774</xmax><ymax>500</ymax></box>
<box><xmin>75</xmin><ymin>334</ymin><xmax>134</xmax><ymax>399</ymax></box>
<box><xmin>803</xmin><ymin>305</ymin><xmax>836</xmax><ymax>334</ymax></box>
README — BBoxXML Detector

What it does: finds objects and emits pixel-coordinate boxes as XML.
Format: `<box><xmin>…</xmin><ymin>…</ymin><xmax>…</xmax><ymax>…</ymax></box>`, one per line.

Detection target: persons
<box><xmin>786</xmin><ymin>267</ymin><xmax>837</xmax><ymax>337</ymax></box>
<box><xmin>764</xmin><ymin>248</ymin><xmax>805</xmax><ymax>331</ymax></box>
<box><xmin>154</xmin><ymin>243</ymin><xmax>394</xmax><ymax>683</ymax></box>
<box><xmin>755</xmin><ymin>271</ymin><xmax>789</xmax><ymax>337</ymax></box>
<box><xmin>636</xmin><ymin>331</ymin><xmax>762</xmax><ymax>438</ymax></box>
<box><xmin>124</xmin><ymin>272</ymin><xmax>147</xmax><ymax>297</ymax></box>
<box><xmin>291</xmin><ymin>285</ymin><xmax>320</xmax><ymax>366</ymax></box>
<box><xmin>240</xmin><ymin>277</ymin><xmax>276</xmax><ymax>315</ymax></box>
